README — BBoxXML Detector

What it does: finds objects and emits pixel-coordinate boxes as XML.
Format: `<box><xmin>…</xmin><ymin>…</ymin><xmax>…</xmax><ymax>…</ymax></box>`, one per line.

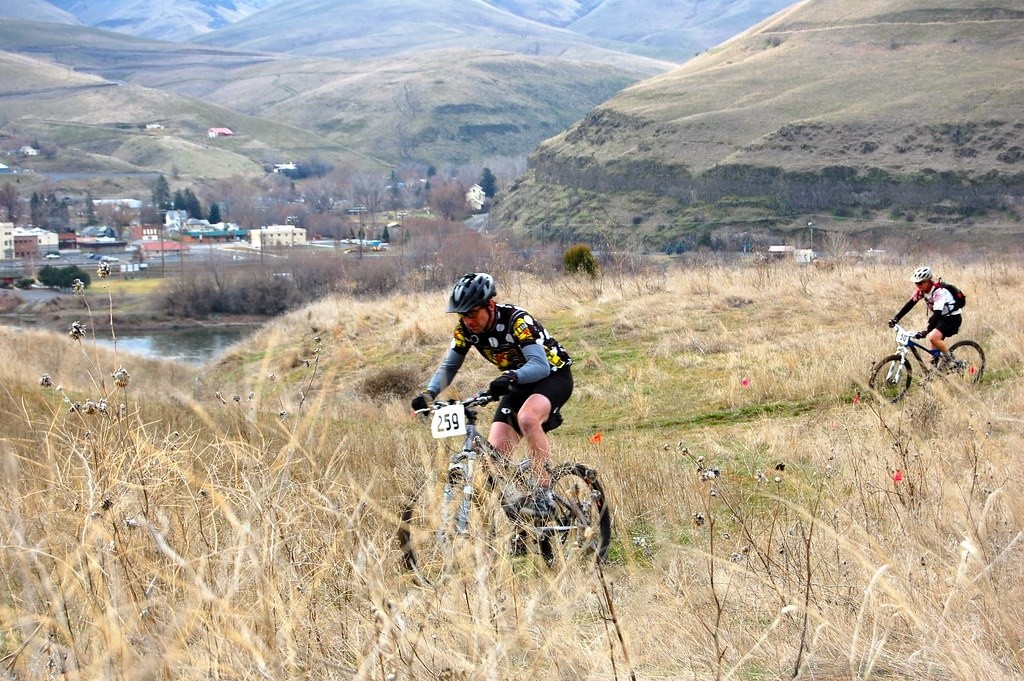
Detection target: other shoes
<box><xmin>938</xmin><ymin>353</ymin><xmax>955</xmax><ymax>370</ymax></box>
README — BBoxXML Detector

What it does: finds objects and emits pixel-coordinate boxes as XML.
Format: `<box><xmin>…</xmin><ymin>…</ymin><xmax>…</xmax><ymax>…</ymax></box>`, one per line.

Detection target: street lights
<box><xmin>808</xmin><ymin>222</ymin><xmax>814</xmax><ymax>249</ymax></box>
<box><xmin>160</xmin><ymin>223</ymin><xmax>170</xmax><ymax>278</ymax></box>
<box><xmin>291</xmin><ymin>227</ymin><xmax>296</xmax><ymax>257</ymax></box>
<box><xmin>260</xmin><ymin>226</ymin><xmax>265</xmax><ymax>263</ymax></box>
<box><xmin>397</xmin><ymin>212</ymin><xmax>407</xmax><ymax>254</ymax></box>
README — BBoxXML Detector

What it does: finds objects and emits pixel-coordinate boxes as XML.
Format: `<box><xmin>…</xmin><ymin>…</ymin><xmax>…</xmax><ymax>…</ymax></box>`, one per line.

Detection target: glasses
<box><xmin>915</xmin><ymin>281</ymin><xmax>923</xmax><ymax>285</ymax></box>
<box><xmin>458</xmin><ymin>306</ymin><xmax>484</xmax><ymax>319</ymax></box>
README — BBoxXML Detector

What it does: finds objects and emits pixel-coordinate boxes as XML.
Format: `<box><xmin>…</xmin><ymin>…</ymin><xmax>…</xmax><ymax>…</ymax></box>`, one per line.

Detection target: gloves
<box><xmin>489</xmin><ymin>371</ymin><xmax>518</xmax><ymax>402</ymax></box>
<box><xmin>888</xmin><ymin>319</ymin><xmax>897</xmax><ymax>328</ymax></box>
<box><xmin>412</xmin><ymin>395</ymin><xmax>435</xmax><ymax>416</ymax></box>
<box><xmin>915</xmin><ymin>330</ymin><xmax>927</xmax><ymax>339</ymax></box>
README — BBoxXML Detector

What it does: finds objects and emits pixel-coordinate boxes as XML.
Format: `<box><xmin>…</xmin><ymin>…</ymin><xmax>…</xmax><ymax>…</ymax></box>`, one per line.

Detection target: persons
<box><xmin>411</xmin><ymin>271</ymin><xmax>575</xmax><ymax>554</ymax></box>
<box><xmin>888</xmin><ymin>266</ymin><xmax>963</xmax><ymax>385</ymax></box>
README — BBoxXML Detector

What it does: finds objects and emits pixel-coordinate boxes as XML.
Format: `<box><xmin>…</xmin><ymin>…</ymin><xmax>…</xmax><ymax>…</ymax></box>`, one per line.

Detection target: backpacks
<box><xmin>932</xmin><ymin>280</ymin><xmax>966</xmax><ymax>315</ymax></box>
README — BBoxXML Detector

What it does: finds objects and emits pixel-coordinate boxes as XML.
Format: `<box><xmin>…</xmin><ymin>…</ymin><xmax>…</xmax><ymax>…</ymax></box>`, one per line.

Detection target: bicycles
<box><xmin>396</xmin><ymin>382</ymin><xmax>611</xmax><ymax>595</ymax></box>
<box><xmin>867</xmin><ymin>321</ymin><xmax>986</xmax><ymax>407</ymax></box>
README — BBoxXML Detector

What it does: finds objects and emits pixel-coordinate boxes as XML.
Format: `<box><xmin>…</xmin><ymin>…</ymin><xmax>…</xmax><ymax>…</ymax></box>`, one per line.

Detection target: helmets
<box><xmin>445</xmin><ymin>272</ymin><xmax>497</xmax><ymax>313</ymax></box>
<box><xmin>909</xmin><ymin>267</ymin><xmax>932</xmax><ymax>282</ymax></box>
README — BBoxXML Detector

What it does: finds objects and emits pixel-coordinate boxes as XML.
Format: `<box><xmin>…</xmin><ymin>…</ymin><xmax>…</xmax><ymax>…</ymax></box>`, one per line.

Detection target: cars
<box><xmin>46</xmin><ymin>253</ymin><xmax>61</xmax><ymax>259</ymax></box>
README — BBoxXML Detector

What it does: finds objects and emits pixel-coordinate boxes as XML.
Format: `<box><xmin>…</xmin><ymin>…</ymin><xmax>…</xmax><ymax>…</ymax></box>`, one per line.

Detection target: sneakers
<box><xmin>520</xmin><ymin>494</ymin><xmax>553</xmax><ymax>517</ymax></box>
